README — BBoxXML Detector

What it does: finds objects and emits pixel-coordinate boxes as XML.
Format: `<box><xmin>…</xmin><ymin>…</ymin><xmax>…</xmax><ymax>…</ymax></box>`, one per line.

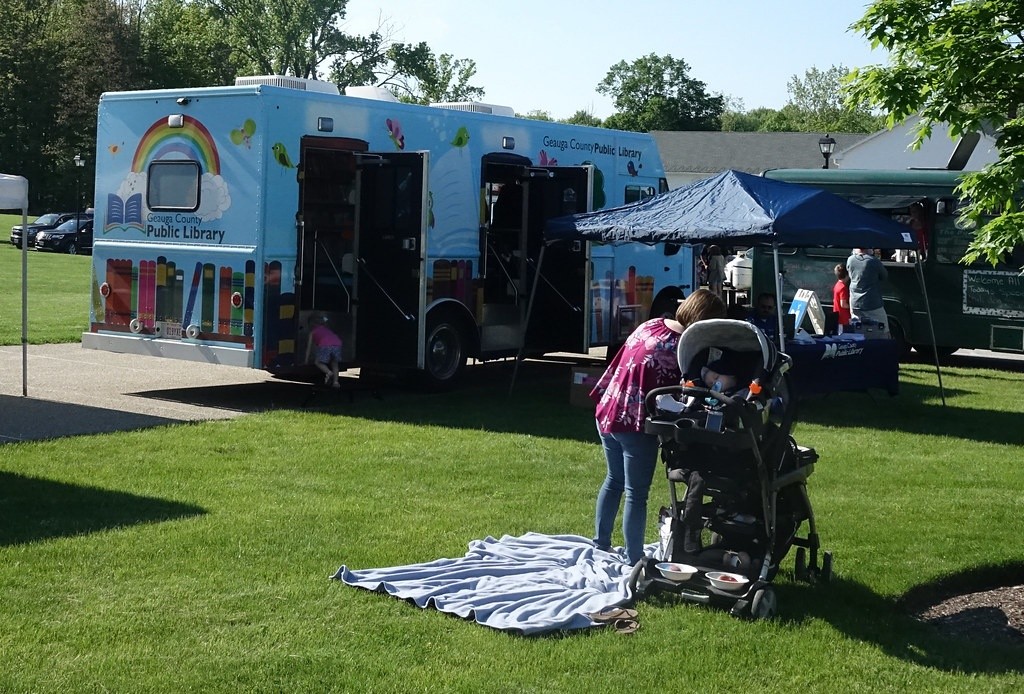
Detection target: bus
<box><xmin>748</xmin><ymin>167</ymin><xmax>1024</xmax><ymax>367</ymax></box>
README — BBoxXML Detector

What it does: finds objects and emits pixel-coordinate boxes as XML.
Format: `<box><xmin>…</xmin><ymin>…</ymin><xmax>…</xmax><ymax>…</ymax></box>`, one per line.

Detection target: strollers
<box><xmin>630</xmin><ymin>319</ymin><xmax>834</xmax><ymax>624</ymax></box>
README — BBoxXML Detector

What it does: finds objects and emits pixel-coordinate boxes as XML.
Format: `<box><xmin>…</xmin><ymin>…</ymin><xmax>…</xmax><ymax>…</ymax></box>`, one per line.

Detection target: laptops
<box><xmin>812</xmin><ymin>310</ymin><xmax>839</xmax><ymax>338</ymax></box>
<box><xmin>772</xmin><ymin>314</ymin><xmax>797</xmax><ymax>342</ymax></box>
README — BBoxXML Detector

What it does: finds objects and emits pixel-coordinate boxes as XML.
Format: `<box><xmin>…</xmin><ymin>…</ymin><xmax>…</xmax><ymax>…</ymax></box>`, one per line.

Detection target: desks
<box><xmin>776</xmin><ymin>335</ymin><xmax>898</xmax><ymax>398</ymax></box>
<box><xmin>699</xmin><ymin>285</ymin><xmax>751</xmax><ymax>308</ymax></box>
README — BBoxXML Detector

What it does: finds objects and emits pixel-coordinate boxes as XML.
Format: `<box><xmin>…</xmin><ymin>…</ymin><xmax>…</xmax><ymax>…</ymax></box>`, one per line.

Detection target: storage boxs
<box><xmin>571</xmin><ymin>366</ymin><xmax>608</xmax><ymax>408</ymax></box>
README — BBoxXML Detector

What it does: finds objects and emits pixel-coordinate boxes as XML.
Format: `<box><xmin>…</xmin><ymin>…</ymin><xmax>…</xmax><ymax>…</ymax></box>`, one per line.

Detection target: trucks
<box><xmin>81</xmin><ymin>76</ymin><xmax>703</xmax><ymax>404</ymax></box>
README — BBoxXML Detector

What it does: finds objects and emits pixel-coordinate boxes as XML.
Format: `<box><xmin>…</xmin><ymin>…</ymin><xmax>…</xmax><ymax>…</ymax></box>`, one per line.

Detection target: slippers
<box><xmin>589</xmin><ymin>606</ymin><xmax>638</xmax><ymax>622</ymax></box>
<box><xmin>615</xmin><ymin>620</ymin><xmax>640</xmax><ymax>634</ymax></box>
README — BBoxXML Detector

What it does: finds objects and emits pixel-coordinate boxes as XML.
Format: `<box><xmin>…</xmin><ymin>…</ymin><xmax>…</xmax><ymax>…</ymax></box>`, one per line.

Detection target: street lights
<box><xmin>73</xmin><ymin>153</ymin><xmax>86</xmax><ymax>255</ymax></box>
<box><xmin>818</xmin><ymin>133</ymin><xmax>836</xmax><ymax>170</ymax></box>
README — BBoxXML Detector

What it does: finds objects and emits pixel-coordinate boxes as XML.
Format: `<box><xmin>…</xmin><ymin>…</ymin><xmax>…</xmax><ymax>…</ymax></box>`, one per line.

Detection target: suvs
<box><xmin>10</xmin><ymin>212</ymin><xmax>94</xmax><ymax>250</ymax></box>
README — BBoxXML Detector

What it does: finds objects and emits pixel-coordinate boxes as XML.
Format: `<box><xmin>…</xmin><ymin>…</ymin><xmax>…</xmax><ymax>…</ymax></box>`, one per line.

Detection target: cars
<box><xmin>35</xmin><ymin>219</ymin><xmax>92</xmax><ymax>256</ymax></box>
<box><xmin>724</xmin><ymin>247</ymin><xmax>754</xmax><ymax>290</ymax></box>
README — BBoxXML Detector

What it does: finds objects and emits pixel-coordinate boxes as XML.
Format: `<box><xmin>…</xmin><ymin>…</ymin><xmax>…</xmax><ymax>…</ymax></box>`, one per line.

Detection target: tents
<box><xmin>509</xmin><ymin>170</ymin><xmax>946</xmax><ymax>406</ymax></box>
<box><xmin>0</xmin><ymin>173</ymin><xmax>29</xmax><ymax>396</ymax></box>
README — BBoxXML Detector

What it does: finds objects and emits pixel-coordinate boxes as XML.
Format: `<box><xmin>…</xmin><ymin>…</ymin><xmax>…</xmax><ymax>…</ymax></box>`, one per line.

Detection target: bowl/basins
<box><xmin>704</xmin><ymin>571</ymin><xmax>749</xmax><ymax>591</ymax></box>
<box><xmin>655</xmin><ymin>562</ymin><xmax>698</xmax><ymax>581</ymax></box>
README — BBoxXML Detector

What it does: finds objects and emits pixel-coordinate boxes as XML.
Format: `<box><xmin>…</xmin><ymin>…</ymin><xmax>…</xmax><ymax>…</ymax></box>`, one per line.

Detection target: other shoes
<box><xmin>334</xmin><ymin>382</ymin><xmax>340</xmax><ymax>388</ymax></box>
<box><xmin>324</xmin><ymin>371</ymin><xmax>333</xmax><ymax>385</ymax></box>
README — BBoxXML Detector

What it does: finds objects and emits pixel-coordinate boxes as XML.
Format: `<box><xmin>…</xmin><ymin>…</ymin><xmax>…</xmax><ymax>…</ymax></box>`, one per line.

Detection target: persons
<box><xmin>697</xmin><ymin>261</ymin><xmax>703</xmax><ymax>287</ymax></box>
<box><xmin>680</xmin><ymin>345</ymin><xmax>754</xmax><ymax>470</ymax></box>
<box><xmin>707</xmin><ymin>245</ymin><xmax>726</xmax><ymax>297</ymax></box>
<box><xmin>588</xmin><ymin>289</ymin><xmax>725</xmax><ymax>564</ymax></box>
<box><xmin>891</xmin><ymin>211</ymin><xmax>928</xmax><ymax>260</ymax></box>
<box><xmin>298</xmin><ymin>315</ymin><xmax>343</xmax><ymax>388</ymax></box>
<box><xmin>846</xmin><ymin>249</ymin><xmax>890</xmax><ymax>339</ymax></box>
<box><xmin>834</xmin><ymin>263</ymin><xmax>850</xmax><ymax>335</ymax></box>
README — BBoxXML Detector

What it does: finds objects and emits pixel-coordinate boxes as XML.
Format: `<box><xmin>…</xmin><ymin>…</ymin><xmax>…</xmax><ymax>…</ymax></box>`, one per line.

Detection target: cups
<box><xmin>874</xmin><ymin>249</ymin><xmax>881</xmax><ymax>261</ymax></box>
